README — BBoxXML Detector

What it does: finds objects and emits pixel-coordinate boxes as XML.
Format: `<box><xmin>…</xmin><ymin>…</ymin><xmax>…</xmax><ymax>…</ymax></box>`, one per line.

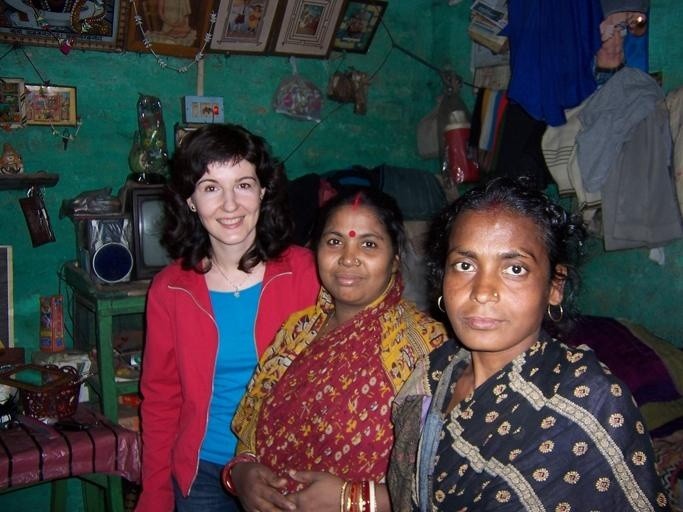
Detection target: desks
<box><xmin>0</xmin><ymin>399</ymin><xmax>128</xmax><ymax>511</ymax></box>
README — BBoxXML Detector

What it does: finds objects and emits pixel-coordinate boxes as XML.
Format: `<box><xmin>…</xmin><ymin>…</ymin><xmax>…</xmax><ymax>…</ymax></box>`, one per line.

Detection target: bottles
<box><xmin>441</xmin><ymin>109</ymin><xmax>481</xmax><ymax>184</ymax></box>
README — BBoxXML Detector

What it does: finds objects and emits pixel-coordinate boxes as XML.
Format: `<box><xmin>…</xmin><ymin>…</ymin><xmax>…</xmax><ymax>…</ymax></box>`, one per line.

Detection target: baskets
<box><xmin>20</xmin><ymin>381</ymin><xmax>81</xmax><ymax>421</ymax></box>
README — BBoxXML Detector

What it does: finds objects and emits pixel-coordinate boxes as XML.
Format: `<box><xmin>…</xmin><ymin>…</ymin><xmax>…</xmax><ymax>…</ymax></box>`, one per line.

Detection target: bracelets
<box><xmin>221</xmin><ymin>454</ymin><xmax>257</xmax><ymax>498</ymax></box>
<box><xmin>340</xmin><ymin>477</ymin><xmax>378</xmax><ymax>511</ymax></box>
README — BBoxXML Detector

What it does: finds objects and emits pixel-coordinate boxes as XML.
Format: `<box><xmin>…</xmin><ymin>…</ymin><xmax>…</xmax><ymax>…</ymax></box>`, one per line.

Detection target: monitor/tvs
<box><xmin>127</xmin><ymin>180</ymin><xmax>177</xmax><ymax>280</ymax></box>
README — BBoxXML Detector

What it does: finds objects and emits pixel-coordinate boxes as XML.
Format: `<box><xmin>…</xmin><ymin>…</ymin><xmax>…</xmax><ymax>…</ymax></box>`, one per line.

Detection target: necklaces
<box><xmin>206</xmin><ymin>252</ymin><xmax>259</xmax><ymax>299</ymax></box>
<box><xmin>314</xmin><ymin>308</ymin><xmax>338</xmax><ymax>343</ymax></box>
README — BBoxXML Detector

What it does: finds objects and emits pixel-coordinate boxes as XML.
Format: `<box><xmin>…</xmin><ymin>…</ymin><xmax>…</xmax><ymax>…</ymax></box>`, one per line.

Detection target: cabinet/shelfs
<box><xmin>70</xmin><ymin>270</ymin><xmax>155</xmax><ymax>511</ymax></box>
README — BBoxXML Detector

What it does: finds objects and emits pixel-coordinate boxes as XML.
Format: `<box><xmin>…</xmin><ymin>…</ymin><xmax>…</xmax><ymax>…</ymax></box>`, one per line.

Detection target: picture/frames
<box><xmin>332</xmin><ymin>0</ymin><xmax>389</xmax><ymax>55</ymax></box>
<box><xmin>206</xmin><ymin>0</ymin><xmax>283</xmax><ymax>57</ymax></box>
<box><xmin>184</xmin><ymin>96</ymin><xmax>225</xmax><ymax>125</ymax></box>
<box><xmin>1</xmin><ymin>1</ymin><xmax>126</xmax><ymax>54</ymax></box>
<box><xmin>25</xmin><ymin>84</ymin><xmax>77</xmax><ymax>127</ymax></box>
<box><xmin>269</xmin><ymin>0</ymin><xmax>349</xmax><ymax>59</ymax></box>
<box><xmin>124</xmin><ymin>0</ymin><xmax>215</xmax><ymax>59</ymax></box>
<box><xmin>0</xmin><ymin>78</ymin><xmax>25</xmax><ymax>129</ymax></box>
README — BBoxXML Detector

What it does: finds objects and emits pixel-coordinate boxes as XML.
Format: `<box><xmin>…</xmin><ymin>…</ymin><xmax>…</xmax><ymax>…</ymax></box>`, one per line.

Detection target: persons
<box><xmin>156</xmin><ymin>0</ymin><xmax>194</xmax><ymax>37</ymax></box>
<box><xmin>383</xmin><ymin>170</ymin><xmax>672</xmax><ymax>512</ymax></box>
<box><xmin>235</xmin><ymin>0</ymin><xmax>262</xmax><ymax>36</ymax></box>
<box><xmin>219</xmin><ymin>185</ymin><xmax>448</xmax><ymax>512</ymax></box>
<box><xmin>133</xmin><ymin>118</ymin><xmax>321</xmax><ymax>511</ymax></box>
